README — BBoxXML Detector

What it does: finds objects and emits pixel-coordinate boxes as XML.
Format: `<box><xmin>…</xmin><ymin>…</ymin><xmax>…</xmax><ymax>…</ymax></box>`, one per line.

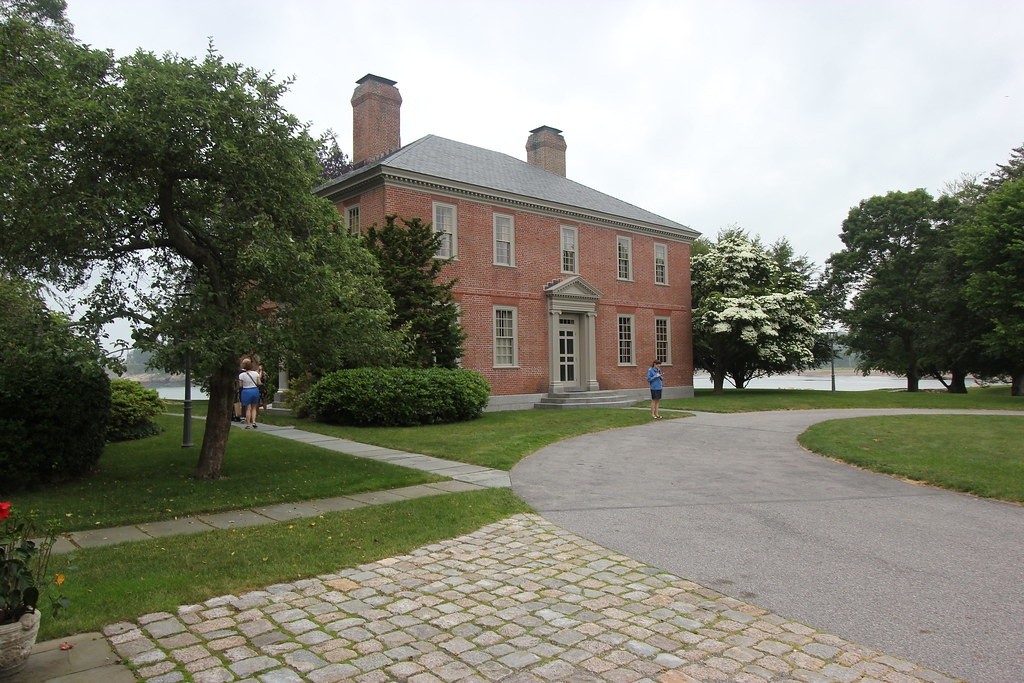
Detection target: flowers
<box><xmin>0</xmin><ymin>502</ymin><xmax>69</xmax><ymax>625</ymax></box>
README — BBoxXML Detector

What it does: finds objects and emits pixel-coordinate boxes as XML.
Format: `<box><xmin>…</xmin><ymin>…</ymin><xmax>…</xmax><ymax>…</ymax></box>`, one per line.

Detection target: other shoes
<box><xmin>245</xmin><ymin>426</ymin><xmax>251</xmax><ymax>429</ymax></box>
<box><xmin>252</xmin><ymin>423</ymin><xmax>258</xmax><ymax>428</ymax></box>
<box><xmin>652</xmin><ymin>415</ymin><xmax>663</xmax><ymax>418</ymax></box>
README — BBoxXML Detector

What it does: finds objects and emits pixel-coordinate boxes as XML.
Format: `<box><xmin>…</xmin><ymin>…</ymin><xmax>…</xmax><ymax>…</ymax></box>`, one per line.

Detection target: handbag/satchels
<box><xmin>234</xmin><ymin>393</ymin><xmax>242</xmax><ymax>417</ymax></box>
<box><xmin>259</xmin><ymin>386</ymin><xmax>267</xmax><ymax>399</ymax></box>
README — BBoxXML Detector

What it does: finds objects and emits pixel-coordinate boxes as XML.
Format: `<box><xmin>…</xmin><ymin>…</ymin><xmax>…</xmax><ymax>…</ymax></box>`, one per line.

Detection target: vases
<box><xmin>0</xmin><ymin>606</ymin><xmax>41</xmax><ymax>678</ymax></box>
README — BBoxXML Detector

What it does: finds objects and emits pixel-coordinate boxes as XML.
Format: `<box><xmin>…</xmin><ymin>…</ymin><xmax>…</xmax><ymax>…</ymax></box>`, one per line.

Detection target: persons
<box><xmin>647</xmin><ymin>360</ymin><xmax>664</xmax><ymax>419</ymax></box>
<box><xmin>238</xmin><ymin>354</ymin><xmax>269</xmax><ymax>429</ymax></box>
